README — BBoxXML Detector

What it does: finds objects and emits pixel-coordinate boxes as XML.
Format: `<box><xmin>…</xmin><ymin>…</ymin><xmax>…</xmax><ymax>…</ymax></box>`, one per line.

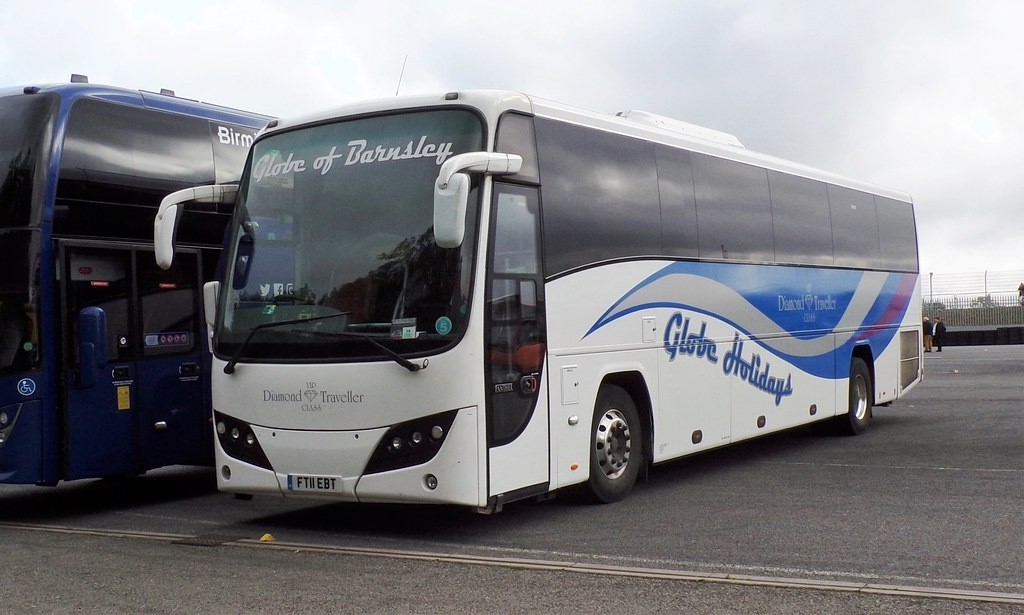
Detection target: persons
<box><xmin>405</xmin><ymin>264</ymin><xmax>452</xmax><ymax>333</ymax></box>
<box><xmin>932</xmin><ymin>317</ymin><xmax>943</xmax><ymax>352</ymax></box>
<box><xmin>1018</xmin><ymin>282</ymin><xmax>1024</xmax><ymax>306</ymax></box>
<box><xmin>923</xmin><ymin>317</ymin><xmax>933</xmax><ymax>353</ymax></box>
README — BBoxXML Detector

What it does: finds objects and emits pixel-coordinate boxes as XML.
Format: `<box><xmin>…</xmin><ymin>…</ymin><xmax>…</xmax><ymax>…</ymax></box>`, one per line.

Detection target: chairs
<box><xmin>0</xmin><ymin>310</ymin><xmax>33</xmax><ymax>370</ymax></box>
<box><xmin>325</xmin><ymin>234</ymin><xmax>373</xmax><ymax>324</ymax></box>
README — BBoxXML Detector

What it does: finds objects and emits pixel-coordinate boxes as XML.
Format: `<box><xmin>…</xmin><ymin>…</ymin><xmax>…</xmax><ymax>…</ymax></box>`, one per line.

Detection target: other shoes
<box><xmin>936</xmin><ymin>350</ymin><xmax>941</xmax><ymax>352</ymax></box>
<box><xmin>924</xmin><ymin>350</ymin><xmax>931</xmax><ymax>352</ymax></box>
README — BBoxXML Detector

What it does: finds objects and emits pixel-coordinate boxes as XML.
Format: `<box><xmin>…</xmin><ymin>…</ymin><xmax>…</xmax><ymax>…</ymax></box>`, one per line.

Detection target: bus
<box><xmin>153</xmin><ymin>90</ymin><xmax>925</xmax><ymax>515</ymax></box>
<box><xmin>0</xmin><ymin>73</ymin><xmax>279</xmax><ymax>488</ymax></box>
